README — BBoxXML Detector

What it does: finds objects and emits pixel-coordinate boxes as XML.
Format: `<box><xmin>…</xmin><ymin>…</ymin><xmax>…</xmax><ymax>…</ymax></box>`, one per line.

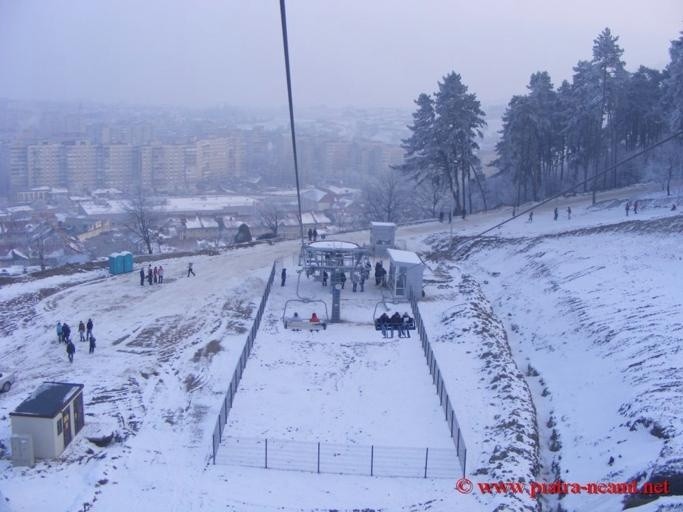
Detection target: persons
<box><xmin>307</xmin><ymin>227</ymin><xmax>312</xmax><ymax>241</ymax></box>
<box><xmin>186</xmin><ymin>262</ymin><xmax>195</xmax><ymax>278</ymax></box>
<box><xmin>308</xmin><ymin>312</ymin><xmax>321</xmax><ymax>332</ymax></box>
<box><xmin>400</xmin><ymin>312</ymin><xmax>411</xmax><ymax>338</ymax></box>
<box><xmin>56</xmin><ymin>318</ymin><xmax>96</xmax><ymax>363</ymax></box>
<box><xmin>312</xmin><ymin>228</ymin><xmax>317</xmax><ymax>242</ymax></box>
<box><xmin>290</xmin><ymin>312</ymin><xmax>301</xmax><ymax>331</ymax></box>
<box><xmin>390</xmin><ymin>311</ymin><xmax>402</xmax><ymax>338</ymax></box>
<box><xmin>303</xmin><ymin>254</ymin><xmax>388</xmax><ymax>293</ymax></box>
<box><xmin>139</xmin><ymin>263</ymin><xmax>164</xmax><ymax>286</ymax></box>
<box><xmin>378</xmin><ymin>313</ymin><xmax>392</xmax><ymax>336</ymax></box>
<box><xmin>279</xmin><ymin>268</ymin><xmax>288</xmax><ymax>287</ymax></box>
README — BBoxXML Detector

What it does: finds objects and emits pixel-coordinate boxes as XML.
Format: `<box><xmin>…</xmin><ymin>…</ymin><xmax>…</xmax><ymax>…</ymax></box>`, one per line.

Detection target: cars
<box><xmin>0</xmin><ymin>371</ymin><xmax>15</xmax><ymax>393</ymax></box>
